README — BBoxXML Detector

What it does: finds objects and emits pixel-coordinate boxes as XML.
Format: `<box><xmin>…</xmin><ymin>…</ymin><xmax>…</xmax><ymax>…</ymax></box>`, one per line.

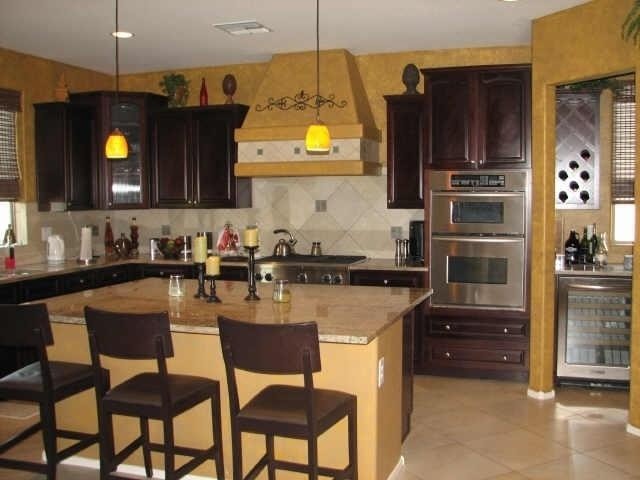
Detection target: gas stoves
<box><xmin>256</xmin><ymin>254</ymin><xmax>366</xmax><ymax>273</ymax></box>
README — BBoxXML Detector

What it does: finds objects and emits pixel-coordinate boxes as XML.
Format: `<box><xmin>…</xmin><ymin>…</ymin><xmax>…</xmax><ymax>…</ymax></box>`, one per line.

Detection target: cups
<box><xmin>170</xmin><ymin>274</ymin><xmax>189</xmax><ymax>297</ymax></box>
<box><xmin>272</xmin><ymin>277</ymin><xmax>294</xmax><ymax>303</ymax></box>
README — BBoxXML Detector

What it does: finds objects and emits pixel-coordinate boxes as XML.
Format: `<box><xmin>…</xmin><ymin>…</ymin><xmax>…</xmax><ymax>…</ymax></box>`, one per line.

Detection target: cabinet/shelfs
<box><xmin>420</xmin><ymin>63</ymin><xmax>532</xmax><ymax>168</ymax></box>
<box><xmin>384</xmin><ymin>94</ymin><xmax>425</xmax><ymax>209</ymax></box>
<box><xmin>422</xmin><ymin>308</ymin><xmax>530</xmax><ymax>384</ymax></box>
<box><xmin>34</xmin><ymin>102</ymin><xmax>93</xmax><ymax>211</ymax></box>
<box><xmin>71</xmin><ymin>91</ymin><xmax>168</xmax><ymax>210</ymax></box>
<box><xmin>1</xmin><ymin>265</ymin><xmax>131</xmax><ymax>381</ymax></box>
<box><xmin>350</xmin><ymin>269</ymin><xmax>429</xmax><ymax>377</ymax></box>
<box><xmin>152</xmin><ymin>104</ymin><xmax>252</xmax><ymax>210</ymax></box>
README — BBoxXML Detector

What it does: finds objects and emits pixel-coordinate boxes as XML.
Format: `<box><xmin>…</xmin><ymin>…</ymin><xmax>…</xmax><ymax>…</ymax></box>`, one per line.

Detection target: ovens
<box><xmin>423</xmin><ymin>167</ymin><xmax>528</xmax><ymax>315</ymax></box>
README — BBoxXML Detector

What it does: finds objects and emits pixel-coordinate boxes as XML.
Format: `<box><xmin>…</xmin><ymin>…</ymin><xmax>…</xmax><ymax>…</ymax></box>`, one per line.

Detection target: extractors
<box><xmin>233</xmin><ymin>49</ymin><xmax>384</xmax><ymax>178</ymax></box>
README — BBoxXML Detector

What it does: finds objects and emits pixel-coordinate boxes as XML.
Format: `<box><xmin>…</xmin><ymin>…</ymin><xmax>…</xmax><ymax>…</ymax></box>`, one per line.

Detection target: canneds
<box><xmin>311</xmin><ymin>241</ymin><xmax>321</xmax><ymax>257</ymax></box>
<box><xmin>272</xmin><ymin>278</ymin><xmax>291</xmax><ymax>304</ymax></box>
<box><xmin>168</xmin><ymin>273</ymin><xmax>186</xmax><ymax>297</ymax></box>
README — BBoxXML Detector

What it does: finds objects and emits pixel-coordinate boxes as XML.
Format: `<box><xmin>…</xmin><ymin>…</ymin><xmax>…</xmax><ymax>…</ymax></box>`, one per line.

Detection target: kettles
<box><xmin>274</xmin><ymin>238</ymin><xmax>291</xmax><ymax>259</ymax></box>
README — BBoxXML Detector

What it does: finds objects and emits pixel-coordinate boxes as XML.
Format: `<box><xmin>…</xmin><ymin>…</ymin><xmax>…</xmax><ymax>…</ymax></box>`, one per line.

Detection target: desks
<box><xmin>19</xmin><ymin>275</ymin><xmax>435</xmax><ymax>480</ymax></box>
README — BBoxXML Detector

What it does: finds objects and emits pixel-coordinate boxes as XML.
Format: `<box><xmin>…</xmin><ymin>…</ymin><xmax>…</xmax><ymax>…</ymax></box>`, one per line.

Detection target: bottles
<box><xmin>103</xmin><ymin>215</ymin><xmax>115</xmax><ymax>255</ymax></box>
<box><xmin>396</xmin><ymin>238</ymin><xmax>402</xmax><ymax>258</ymax></box>
<box><xmin>565</xmin><ymin>228</ymin><xmax>580</xmax><ymax>266</ymax></box>
<box><xmin>595</xmin><ymin>231</ymin><xmax>608</xmax><ymax>265</ymax></box>
<box><xmin>129</xmin><ymin>216</ymin><xmax>140</xmax><ymax>250</ymax></box>
<box><xmin>199</xmin><ymin>76</ymin><xmax>209</xmax><ymax>106</ymax></box>
<box><xmin>402</xmin><ymin>239</ymin><xmax>409</xmax><ymax>260</ymax></box>
<box><xmin>589</xmin><ymin>222</ymin><xmax>598</xmax><ymax>264</ymax></box>
<box><xmin>579</xmin><ymin>223</ymin><xmax>589</xmax><ymax>265</ymax></box>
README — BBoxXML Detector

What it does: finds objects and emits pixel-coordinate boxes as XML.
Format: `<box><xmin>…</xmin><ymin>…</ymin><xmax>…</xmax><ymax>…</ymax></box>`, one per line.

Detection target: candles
<box><xmin>205</xmin><ymin>255</ymin><xmax>220</xmax><ymax>274</ymax></box>
<box><xmin>194</xmin><ymin>236</ymin><xmax>207</xmax><ymax>263</ymax></box>
<box><xmin>244</xmin><ymin>225</ymin><xmax>258</xmax><ymax>247</ymax></box>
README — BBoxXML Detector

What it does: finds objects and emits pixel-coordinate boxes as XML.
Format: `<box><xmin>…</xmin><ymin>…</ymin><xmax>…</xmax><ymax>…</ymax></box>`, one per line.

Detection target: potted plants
<box><xmin>158</xmin><ymin>72</ymin><xmax>189</xmax><ymax>108</ymax></box>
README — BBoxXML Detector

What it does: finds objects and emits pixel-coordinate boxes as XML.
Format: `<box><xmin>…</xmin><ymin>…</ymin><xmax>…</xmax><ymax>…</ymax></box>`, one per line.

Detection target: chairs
<box><xmin>83</xmin><ymin>306</ymin><xmax>224</xmax><ymax>480</ymax></box>
<box><xmin>1</xmin><ymin>302</ymin><xmax>117</xmax><ymax>479</ymax></box>
<box><xmin>217</xmin><ymin>314</ymin><xmax>359</xmax><ymax>479</ymax></box>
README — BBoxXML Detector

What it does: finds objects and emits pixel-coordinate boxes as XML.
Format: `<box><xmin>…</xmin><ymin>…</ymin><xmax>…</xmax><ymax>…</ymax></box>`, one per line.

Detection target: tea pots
<box><xmin>46</xmin><ymin>235</ymin><xmax>68</xmax><ymax>263</ymax></box>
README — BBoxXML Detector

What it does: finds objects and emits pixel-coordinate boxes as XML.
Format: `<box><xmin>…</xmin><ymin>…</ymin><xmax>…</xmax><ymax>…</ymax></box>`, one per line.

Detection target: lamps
<box><xmin>305</xmin><ymin>0</ymin><xmax>332</xmax><ymax>152</ymax></box>
<box><xmin>105</xmin><ymin>0</ymin><xmax>128</xmax><ymax>158</ymax></box>
